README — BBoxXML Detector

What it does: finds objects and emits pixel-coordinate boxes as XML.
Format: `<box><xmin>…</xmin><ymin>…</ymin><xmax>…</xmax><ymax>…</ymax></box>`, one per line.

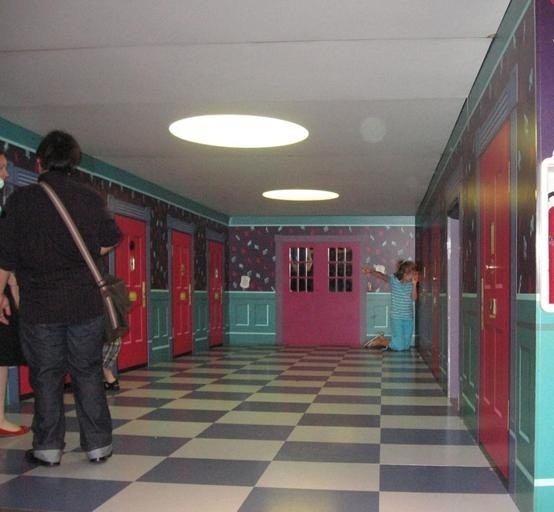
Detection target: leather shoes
<box><xmin>90</xmin><ymin>451</ymin><xmax>113</xmax><ymax>462</ymax></box>
<box><xmin>104</xmin><ymin>379</ymin><xmax>119</xmax><ymax>390</ymax></box>
<box><xmin>25</xmin><ymin>449</ymin><xmax>60</xmax><ymax>468</ymax></box>
<box><xmin>0</xmin><ymin>425</ymin><xmax>31</xmax><ymax>438</ymax></box>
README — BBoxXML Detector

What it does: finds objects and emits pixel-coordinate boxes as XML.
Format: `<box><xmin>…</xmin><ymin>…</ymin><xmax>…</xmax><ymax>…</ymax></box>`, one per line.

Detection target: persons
<box><xmin>1</xmin><ymin>130</ymin><xmax>126</xmax><ymax>470</ymax></box>
<box><xmin>364</xmin><ymin>259</ymin><xmax>420</xmax><ymax>350</ymax></box>
<box><xmin>0</xmin><ymin>147</ymin><xmax>35</xmax><ymax>434</ymax></box>
<box><xmin>100</xmin><ymin>273</ymin><xmax>123</xmax><ymax>391</ymax></box>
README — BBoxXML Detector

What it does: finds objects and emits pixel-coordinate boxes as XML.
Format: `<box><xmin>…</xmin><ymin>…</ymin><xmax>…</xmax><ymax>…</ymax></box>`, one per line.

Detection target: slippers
<box><xmin>362</xmin><ymin>334</ymin><xmax>384</xmax><ymax>349</ymax></box>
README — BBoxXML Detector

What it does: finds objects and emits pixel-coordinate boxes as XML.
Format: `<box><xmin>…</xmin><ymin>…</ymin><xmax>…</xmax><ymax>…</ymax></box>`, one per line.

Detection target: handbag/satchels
<box><xmin>98</xmin><ymin>273</ymin><xmax>131</xmax><ymax>344</ymax></box>
